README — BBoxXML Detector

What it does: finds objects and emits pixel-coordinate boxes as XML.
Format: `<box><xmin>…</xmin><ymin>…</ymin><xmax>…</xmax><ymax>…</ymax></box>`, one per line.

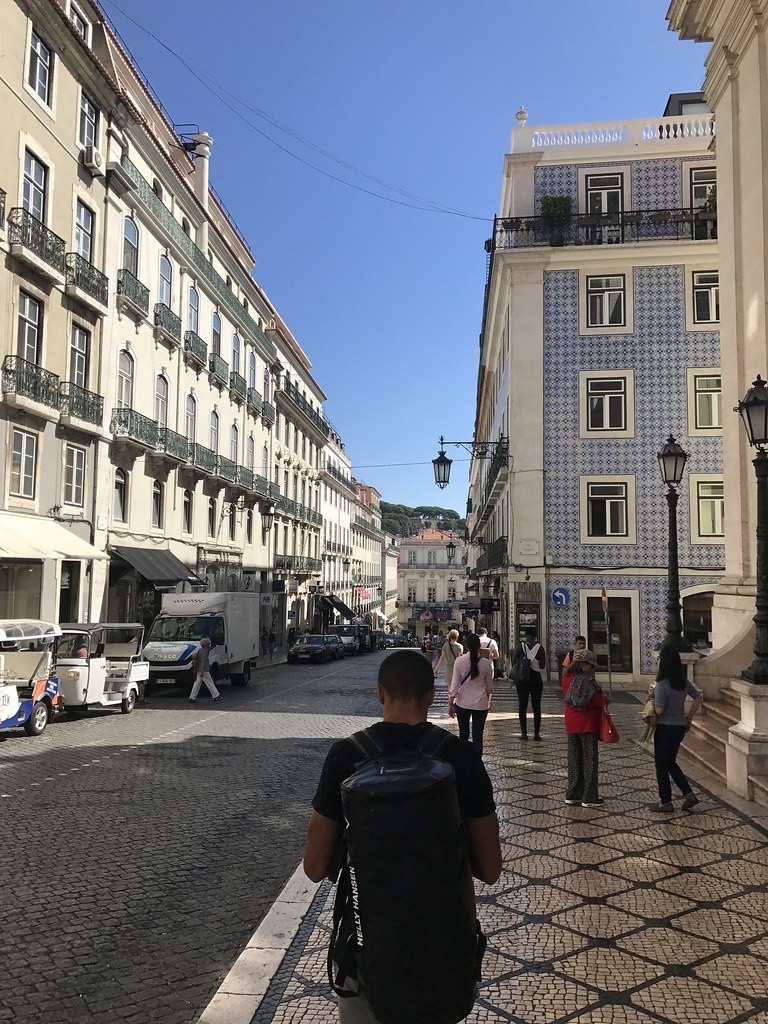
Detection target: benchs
<box><xmin>0</xmin><ymin>651</ymin><xmax>52</xmax><ymax>687</ymax></box>
<box><xmin>102</xmin><ymin>642</ymin><xmax>138</xmax><ymax>674</ymax></box>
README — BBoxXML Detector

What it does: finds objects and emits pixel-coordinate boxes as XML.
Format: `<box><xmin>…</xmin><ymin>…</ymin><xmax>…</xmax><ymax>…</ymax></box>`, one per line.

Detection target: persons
<box><xmin>407</xmin><ymin>632</ymin><xmax>413</xmax><ymax>647</ymax></box>
<box><xmin>562</xmin><ymin>649</ymin><xmax>608</xmax><ymax>807</ymax></box>
<box><xmin>304</xmin><ymin>650</ymin><xmax>502</xmax><ymax>1024</ymax></box>
<box><xmin>422</xmin><ymin>622</ymin><xmax>503</xmax><ymax>681</ymax></box>
<box><xmin>188</xmin><ymin>638</ymin><xmax>224</xmax><ymax>703</ymax></box>
<box><xmin>511</xmin><ymin>627</ymin><xmax>546</xmax><ymax>741</ymax></box>
<box><xmin>434</xmin><ymin>629</ymin><xmax>464</xmax><ymax>719</ymax></box>
<box><xmin>71</xmin><ymin>636</ymin><xmax>88</xmax><ymax>657</ymax></box>
<box><xmin>650</xmin><ymin>646</ymin><xmax>702</xmax><ymax>812</ymax></box>
<box><xmin>477</xmin><ymin>627</ymin><xmax>500</xmax><ymax>711</ymax></box>
<box><xmin>562</xmin><ymin>636</ymin><xmax>586</xmax><ymax>669</ymax></box>
<box><xmin>448</xmin><ymin>634</ymin><xmax>494</xmax><ymax>759</ymax></box>
<box><xmin>128</xmin><ymin>629</ymin><xmax>150</xmax><ymax>705</ymax></box>
<box><xmin>260</xmin><ymin>627</ymin><xmax>312</xmax><ymax>659</ymax></box>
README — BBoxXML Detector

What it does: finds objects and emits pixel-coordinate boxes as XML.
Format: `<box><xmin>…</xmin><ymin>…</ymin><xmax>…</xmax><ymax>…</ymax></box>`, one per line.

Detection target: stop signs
<box><xmin>602</xmin><ymin>586</ymin><xmax>608</xmax><ymax>613</ymax></box>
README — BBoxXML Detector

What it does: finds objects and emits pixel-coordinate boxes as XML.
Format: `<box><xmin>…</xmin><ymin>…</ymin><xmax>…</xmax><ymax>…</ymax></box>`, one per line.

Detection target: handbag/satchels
<box><xmin>509</xmin><ymin>642</ymin><xmax>532</xmax><ymax>681</ymax></box>
<box><xmin>598</xmin><ymin>697</ymin><xmax>619</xmax><ymax>743</ymax></box>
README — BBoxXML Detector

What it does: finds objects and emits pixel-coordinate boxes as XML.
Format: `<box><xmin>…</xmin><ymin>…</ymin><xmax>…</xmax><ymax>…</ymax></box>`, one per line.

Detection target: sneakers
<box><xmin>565</xmin><ymin>797</ymin><xmax>584</xmax><ymax>805</ymax></box>
<box><xmin>583</xmin><ymin>798</ymin><xmax>604</xmax><ymax>807</ymax></box>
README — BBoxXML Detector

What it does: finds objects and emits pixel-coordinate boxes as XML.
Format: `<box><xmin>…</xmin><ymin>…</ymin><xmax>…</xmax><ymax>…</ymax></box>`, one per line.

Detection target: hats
<box><xmin>574</xmin><ymin>649</ymin><xmax>599</xmax><ymax>667</ymax></box>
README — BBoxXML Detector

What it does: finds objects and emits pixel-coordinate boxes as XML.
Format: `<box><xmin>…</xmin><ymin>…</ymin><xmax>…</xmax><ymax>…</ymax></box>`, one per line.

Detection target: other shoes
<box><xmin>533</xmin><ymin>736</ymin><xmax>541</xmax><ymax>741</ymax></box>
<box><xmin>189</xmin><ymin>698</ymin><xmax>199</xmax><ymax>703</ymax></box>
<box><xmin>213</xmin><ymin>696</ymin><xmax>224</xmax><ymax>701</ymax></box>
<box><xmin>519</xmin><ymin>736</ymin><xmax>528</xmax><ymax>740</ymax></box>
<box><xmin>682</xmin><ymin>795</ymin><xmax>699</xmax><ymax>809</ymax></box>
<box><xmin>651</xmin><ymin>803</ymin><xmax>673</xmax><ymax>813</ymax></box>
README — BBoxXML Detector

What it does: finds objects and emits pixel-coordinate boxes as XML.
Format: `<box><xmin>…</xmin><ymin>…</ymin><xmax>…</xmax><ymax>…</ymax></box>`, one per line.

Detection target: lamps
<box><xmin>431</xmin><ymin>434</ymin><xmax>509</xmax><ymax>490</ymax></box>
<box><xmin>222</xmin><ymin>498</ymin><xmax>275</xmax><ymax>532</ymax></box>
<box><xmin>447</xmin><ymin>572</ymin><xmax>469</xmax><ymax>590</ymax></box>
<box><xmin>446</xmin><ymin>536</ymin><xmax>485</xmax><ymax>561</ymax></box>
<box><xmin>397</xmin><ymin>597</ymin><xmax>401</xmax><ymax>603</ymax></box>
<box><xmin>376</xmin><ymin>586</ymin><xmax>383</xmax><ymax>596</ymax></box>
<box><xmin>326</xmin><ymin>555</ymin><xmax>350</xmax><ymax>573</ymax></box>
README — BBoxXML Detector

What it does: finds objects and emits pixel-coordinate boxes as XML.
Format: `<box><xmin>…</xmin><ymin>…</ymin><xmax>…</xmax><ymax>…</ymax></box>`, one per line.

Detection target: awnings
<box><xmin>110</xmin><ymin>545</ymin><xmax>208</xmax><ymax>589</ymax></box>
<box><xmin>355</xmin><ymin>588</ymin><xmax>370</xmax><ymax>597</ymax></box>
<box><xmin>323</xmin><ymin>595</ymin><xmax>356</xmax><ymax>620</ymax></box>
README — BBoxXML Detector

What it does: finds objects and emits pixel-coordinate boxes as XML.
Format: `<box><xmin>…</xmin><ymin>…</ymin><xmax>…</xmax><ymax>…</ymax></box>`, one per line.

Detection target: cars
<box><xmin>287</xmin><ymin>635</ymin><xmax>332</xmax><ymax>665</ymax></box>
<box><xmin>372</xmin><ymin>630</ymin><xmax>405</xmax><ymax>650</ymax></box>
<box><xmin>324</xmin><ymin>635</ymin><xmax>346</xmax><ymax>661</ymax></box>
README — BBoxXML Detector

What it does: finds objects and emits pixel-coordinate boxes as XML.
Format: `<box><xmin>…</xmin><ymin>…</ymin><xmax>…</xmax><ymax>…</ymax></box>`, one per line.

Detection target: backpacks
<box><xmin>191</xmin><ymin>649</ymin><xmax>202</xmax><ymax>673</ymax></box>
<box><xmin>422</xmin><ymin>638</ymin><xmax>432</xmax><ymax>653</ymax></box>
<box><xmin>327</xmin><ymin>727</ymin><xmax>487</xmax><ymax>1024</ymax></box>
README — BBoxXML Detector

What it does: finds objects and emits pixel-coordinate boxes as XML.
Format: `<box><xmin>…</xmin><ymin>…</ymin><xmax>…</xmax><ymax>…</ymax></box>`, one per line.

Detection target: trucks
<box><xmin>331</xmin><ymin>624</ymin><xmax>372</xmax><ymax>657</ymax></box>
<box><xmin>140</xmin><ymin>593</ymin><xmax>260</xmax><ymax>697</ymax></box>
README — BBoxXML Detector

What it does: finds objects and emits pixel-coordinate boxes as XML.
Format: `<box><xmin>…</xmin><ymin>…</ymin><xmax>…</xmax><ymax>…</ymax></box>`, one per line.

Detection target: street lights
<box><xmin>656</xmin><ymin>432</ymin><xmax>694</xmax><ymax>654</ymax></box>
<box><xmin>731</xmin><ymin>372</ymin><xmax>768</xmax><ymax>685</ymax></box>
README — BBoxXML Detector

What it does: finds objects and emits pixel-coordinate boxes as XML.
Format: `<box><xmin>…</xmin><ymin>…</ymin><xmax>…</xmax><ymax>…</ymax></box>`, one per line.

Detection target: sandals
<box><xmin>138</xmin><ymin>700</ymin><xmax>151</xmax><ymax>704</ymax></box>
